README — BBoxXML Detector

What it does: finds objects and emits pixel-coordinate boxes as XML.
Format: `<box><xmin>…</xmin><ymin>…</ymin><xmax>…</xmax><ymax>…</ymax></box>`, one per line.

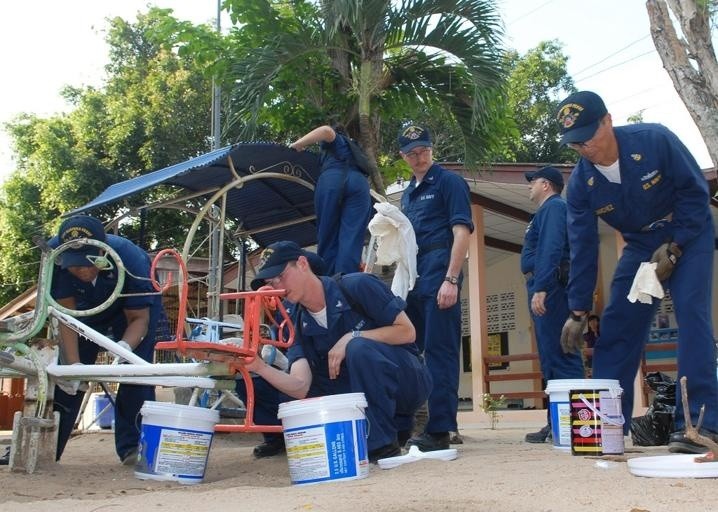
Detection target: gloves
<box><xmin>105</xmin><ymin>340</ymin><xmax>133</xmax><ymax>364</ymax></box>
<box><xmin>58</xmin><ymin>361</ymin><xmax>85</xmax><ymax>397</ymax></box>
<box><xmin>648</xmin><ymin>239</ymin><xmax>684</xmax><ymax>283</ymax></box>
<box><xmin>559</xmin><ymin>312</ymin><xmax>588</xmax><ymax>355</ymax></box>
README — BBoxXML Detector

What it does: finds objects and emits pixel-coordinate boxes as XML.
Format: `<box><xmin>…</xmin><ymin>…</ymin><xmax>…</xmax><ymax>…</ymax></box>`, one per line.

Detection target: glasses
<box><xmin>566</xmin><ymin>139</ymin><xmax>596</xmax><ymax>147</ymax></box>
<box><xmin>527</xmin><ymin>179</ymin><xmax>550</xmax><ymax>186</ymax></box>
<box><xmin>260</xmin><ymin>260</ymin><xmax>296</xmax><ymax>287</ymax></box>
<box><xmin>404</xmin><ymin>147</ymin><xmax>431</xmax><ymax>160</ymax></box>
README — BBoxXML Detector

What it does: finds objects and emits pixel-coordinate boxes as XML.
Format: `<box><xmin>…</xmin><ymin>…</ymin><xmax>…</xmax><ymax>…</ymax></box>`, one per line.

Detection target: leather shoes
<box><xmin>368</xmin><ymin>439</ymin><xmax>401</xmax><ymax>465</ymax></box>
<box><xmin>524</xmin><ymin>422</ymin><xmax>554</xmax><ymax>444</ymax></box>
<box><xmin>668</xmin><ymin>426</ymin><xmax>718</xmax><ymax>455</ymax></box>
<box><xmin>407</xmin><ymin>431</ymin><xmax>450</xmax><ymax>454</ymax></box>
<box><xmin>252</xmin><ymin>441</ymin><xmax>279</xmax><ymax>459</ymax></box>
<box><xmin>449</xmin><ymin>431</ymin><xmax>465</xmax><ymax>446</ymax></box>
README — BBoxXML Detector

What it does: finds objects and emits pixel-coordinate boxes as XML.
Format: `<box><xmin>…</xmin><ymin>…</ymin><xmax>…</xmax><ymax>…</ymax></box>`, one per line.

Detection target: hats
<box><xmin>524</xmin><ymin>166</ymin><xmax>566</xmax><ymax>187</ymax></box>
<box><xmin>58</xmin><ymin>214</ymin><xmax>107</xmax><ymax>271</ymax></box>
<box><xmin>302</xmin><ymin>247</ymin><xmax>329</xmax><ymax>277</ymax></box>
<box><xmin>554</xmin><ymin>91</ymin><xmax>608</xmax><ymax>151</ymax></box>
<box><xmin>248</xmin><ymin>240</ymin><xmax>302</xmax><ymax>290</ymax></box>
<box><xmin>396</xmin><ymin>123</ymin><xmax>432</xmax><ymax>155</ymax></box>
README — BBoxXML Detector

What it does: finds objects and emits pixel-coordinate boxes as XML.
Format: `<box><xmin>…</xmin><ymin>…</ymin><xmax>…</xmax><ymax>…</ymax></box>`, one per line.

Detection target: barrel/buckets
<box><xmin>276</xmin><ymin>392</ymin><xmax>370</xmax><ymax>486</ymax></box>
<box><xmin>132</xmin><ymin>400</ymin><xmax>220</xmax><ymax>485</ymax></box>
<box><xmin>569</xmin><ymin>388</ymin><xmax>625</xmax><ymax>456</ymax></box>
<box><xmin>544</xmin><ymin>379</ymin><xmax>620</xmax><ymax>450</ymax></box>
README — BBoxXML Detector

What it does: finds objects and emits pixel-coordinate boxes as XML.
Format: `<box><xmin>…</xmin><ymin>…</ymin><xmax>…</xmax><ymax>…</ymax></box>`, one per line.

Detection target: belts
<box><xmin>524</xmin><ymin>269</ymin><xmax>540</xmax><ymax>287</ymax></box>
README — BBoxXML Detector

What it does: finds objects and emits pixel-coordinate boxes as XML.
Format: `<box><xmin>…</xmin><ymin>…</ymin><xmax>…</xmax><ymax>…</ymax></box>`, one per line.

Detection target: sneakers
<box><xmin>0</xmin><ymin>446</ymin><xmax>12</xmax><ymax>467</ymax></box>
<box><xmin>124</xmin><ymin>451</ymin><xmax>145</xmax><ymax>468</ymax></box>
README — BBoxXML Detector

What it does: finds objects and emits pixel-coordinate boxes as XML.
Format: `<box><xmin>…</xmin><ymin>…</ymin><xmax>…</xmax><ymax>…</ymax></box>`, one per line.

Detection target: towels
<box><xmin>367</xmin><ymin>200</ymin><xmax>419</xmax><ymax>303</ymax></box>
<box><xmin>626</xmin><ymin>262</ymin><xmax>666</xmax><ymax>307</ymax></box>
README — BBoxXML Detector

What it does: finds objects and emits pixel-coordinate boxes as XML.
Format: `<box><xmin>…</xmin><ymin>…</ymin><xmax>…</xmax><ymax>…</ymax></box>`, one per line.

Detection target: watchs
<box><xmin>444</xmin><ymin>276</ymin><xmax>460</xmax><ymax>285</ymax></box>
<box><xmin>351</xmin><ymin>328</ymin><xmax>360</xmax><ymax>338</ymax></box>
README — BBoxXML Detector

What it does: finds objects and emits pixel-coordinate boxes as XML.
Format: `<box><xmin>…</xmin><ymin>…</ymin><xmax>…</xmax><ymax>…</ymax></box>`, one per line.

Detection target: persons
<box><xmin>584</xmin><ymin>315</ymin><xmax>600</xmax><ymax>369</ymax></box>
<box><xmin>557</xmin><ymin>91</ymin><xmax>718</xmax><ymax>454</ymax></box>
<box><xmin>44</xmin><ymin>214</ymin><xmax>167</xmax><ymax>466</ymax></box>
<box><xmin>396</xmin><ymin>120</ymin><xmax>475</xmax><ymax>452</ymax></box>
<box><xmin>238</xmin><ymin>240</ymin><xmax>433</xmax><ymax>465</ymax></box>
<box><xmin>519</xmin><ymin>167</ymin><xmax>585</xmax><ymax>442</ymax></box>
<box><xmin>287</xmin><ymin>124</ymin><xmax>371</xmax><ymax>274</ymax></box>
<box><xmin>234</xmin><ymin>250</ymin><xmax>329</xmax><ymax>458</ymax></box>
